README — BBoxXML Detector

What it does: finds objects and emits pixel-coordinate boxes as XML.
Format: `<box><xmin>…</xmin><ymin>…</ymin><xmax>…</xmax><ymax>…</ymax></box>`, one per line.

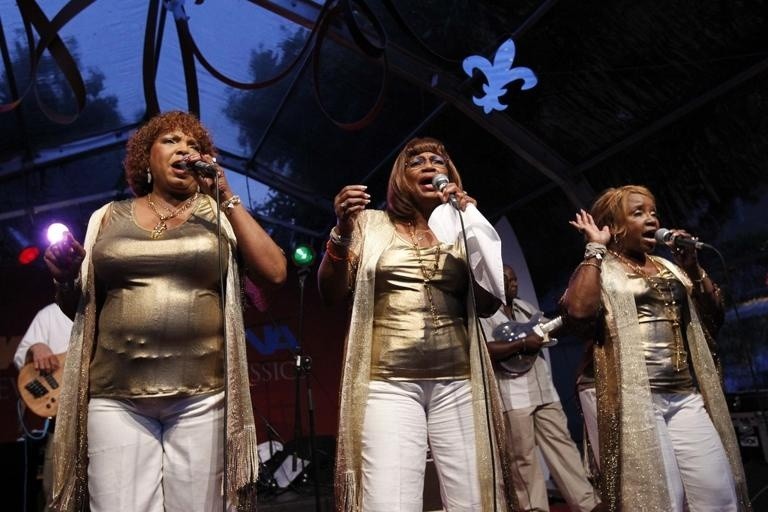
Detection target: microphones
<box><xmin>182</xmin><ymin>155</ymin><xmax>216</xmax><ymax>175</ymax></box>
<box><xmin>432</xmin><ymin>173</ymin><xmax>461</xmax><ymax>211</ymax></box>
<box><xmin>654</xmin><ymin>228</ymin><xmax>714</xmax><ymax>252</ymax></box>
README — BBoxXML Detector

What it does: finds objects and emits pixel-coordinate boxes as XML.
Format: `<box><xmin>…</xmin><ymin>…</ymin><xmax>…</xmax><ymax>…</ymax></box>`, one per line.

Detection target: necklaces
<box><xmin>609</xmin><ymin>250</ymin><xmax>685</xmax><ymax>371</ymax></box>
<box><xmin>146</xmin><ymin>191</ymin><xmax>200</xmax><ymax>239</ymax></box>
<box><xmin>407</xmin><ymin>222</ymin><xmax>440</xmax><ymax>334</ymax></box>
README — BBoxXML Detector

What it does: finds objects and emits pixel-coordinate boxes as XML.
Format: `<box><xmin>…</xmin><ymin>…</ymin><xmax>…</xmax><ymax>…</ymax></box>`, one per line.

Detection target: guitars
<box><xmin>17</xmin><ymin>351</ymin><xmax>69</xmax><ymax>417</ymax></box>
<box><xmin>492</xmin><ymin>310</ymin><xmax>568</xmax><ymax>377</ymax></box>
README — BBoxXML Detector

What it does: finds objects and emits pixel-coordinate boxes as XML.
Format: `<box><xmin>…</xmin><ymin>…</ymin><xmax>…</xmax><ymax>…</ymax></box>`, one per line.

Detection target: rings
<box><xmin>340</xmin><ymin>202</ymin><xmax>346</xmax><ymax>211</ymax></box>
<box><xmin>51</xmin><ymin>245</ymin><xmax>57</xmax><ymax>252</ymax></box>
<box><xmin>212</xmin><ymin>157</ymin><xmax>217</xmax><ymax>162</ymax></box>
<box><xmin>209</xmin><ymin>160</ymin><xmax>214</xmax><ymax>167</ymax></box>
<box><xmin>463</xmin><ymin>190</ymin><xmax>467</xmax><ymax>197</ymax></box>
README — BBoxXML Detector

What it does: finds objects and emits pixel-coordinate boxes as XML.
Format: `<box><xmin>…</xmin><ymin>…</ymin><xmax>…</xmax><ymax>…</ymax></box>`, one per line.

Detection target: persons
<box><xmin>12</xmin><ymin>302</ymin><xmax>74</xmax><ymax>511</ymax></box>
<box><xmin>316</xmin><ymin>136</ymin><xmax>521</xmax><ymax>512</ymax></box>
<box><xmin>44</xmin><ymin>110</ymin><xmax>288</xmax><ymax>512</ymax></box>
<box><xmin>557</xmin><ymin>185</ymin><xmax>751</xmax><ymax>512</ymax></box>
<box><xmin>480</xmin><ymin>264</ymin><xmax>604</xmax><ymax>512</ymax></box>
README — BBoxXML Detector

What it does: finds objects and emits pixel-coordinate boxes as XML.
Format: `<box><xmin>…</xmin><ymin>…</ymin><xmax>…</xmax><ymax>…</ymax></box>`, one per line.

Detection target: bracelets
<box><xmin>584</xmin><ymin>242</ymin><xmax>607</xmax><ymax>260</ymax></box>
<box><xmin>325</xmin><ymin>240</ymin><xmax>350</xmax><ymax>263</ymax></box>
<box><xmin>219</xmin><ymin>195</ymin><xmax>240</xmax><ymax>211</ymax></box>
<box><xmin>52</xmin><ymin>271</ymin><xmax>81</xmax><ymax>293</ymax></box>
<box><xmin>695</xmin><ymin>269</ymin><xmax>706</xmax><ymax>282</ymax></box>
<box><xmin>329</xmin><ymin>226</ymin><xmax>353</xmax><ymax>248</ymax></box>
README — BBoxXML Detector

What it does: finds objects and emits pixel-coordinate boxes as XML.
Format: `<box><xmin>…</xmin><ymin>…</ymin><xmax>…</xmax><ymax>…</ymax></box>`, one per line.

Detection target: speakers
<box><xmin>725</xmin><ymin>389</ymin><xmax>768</xmax><ymax>512</ymax></box>
<box><xmin>0</xmin><ymin>440</ymin><xmax>48</xmax><ymax>512</ymax></box>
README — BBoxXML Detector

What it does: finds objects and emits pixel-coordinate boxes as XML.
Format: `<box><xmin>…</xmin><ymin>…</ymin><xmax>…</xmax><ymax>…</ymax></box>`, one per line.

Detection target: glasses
<box><xmin>406</xmin><ymin>156</ymin><xmax>447</xmax><ymax>169</ymax></box>
